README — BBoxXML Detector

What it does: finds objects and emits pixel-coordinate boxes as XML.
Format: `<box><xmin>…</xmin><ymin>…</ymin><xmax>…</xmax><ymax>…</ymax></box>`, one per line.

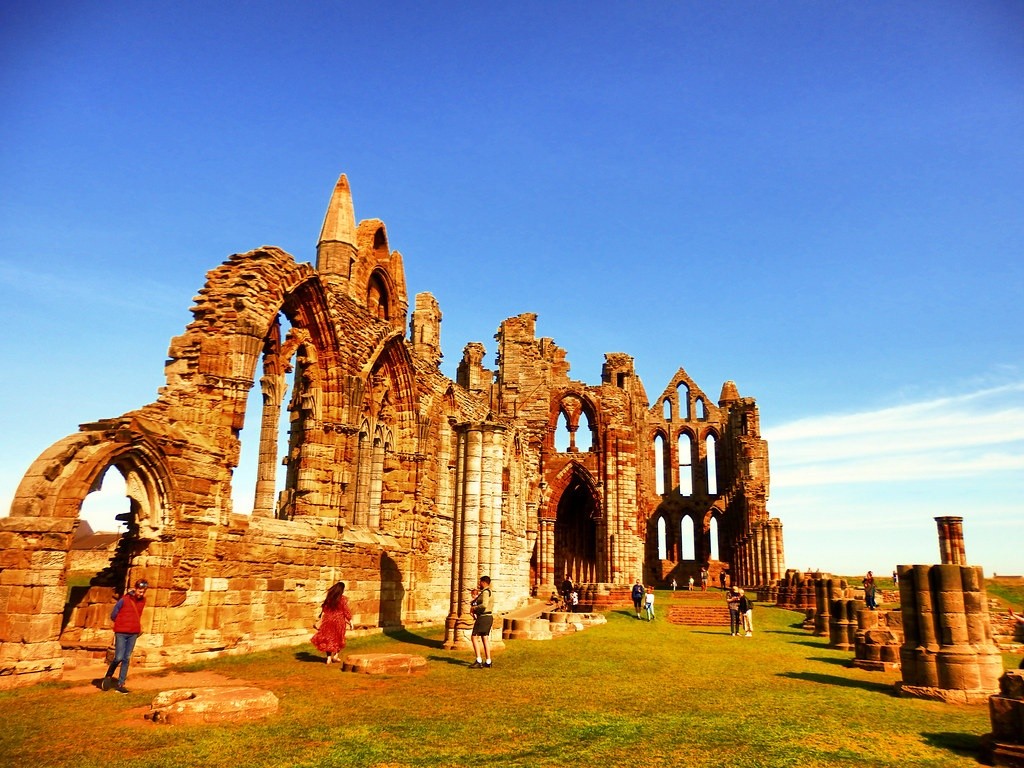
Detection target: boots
<box><xmin>333</xmin><ymin>653</ymin><xmax>341</xmax><ymax>661</ymax></box>
<box><xmin>327</xmin><ymin>655</ymin><xmax>332</xmax><ymax>664</ymax></box>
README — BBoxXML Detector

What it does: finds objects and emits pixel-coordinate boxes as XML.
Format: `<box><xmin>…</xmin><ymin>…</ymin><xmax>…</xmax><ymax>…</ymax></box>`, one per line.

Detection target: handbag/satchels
<box><xmin>744</xmin><ymin>596</ymin><xmax>754</xmax><ymax>609</ymax></box>
<box><xmin>314</xmin><ymin>612</ymin><xmax>325</xmax><ymax>631</ymax></box>
<box><xmin>137</xmin><ymin>624</ymin><xmax>145</xmax><ymax>637</ymax></box>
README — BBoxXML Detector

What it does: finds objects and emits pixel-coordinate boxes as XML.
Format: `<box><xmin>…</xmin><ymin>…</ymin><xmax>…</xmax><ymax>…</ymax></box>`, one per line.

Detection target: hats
<box><xmin>733</xmin><ymin>586</ymin><xmax>738</xmax><ymax>593</ymax></box>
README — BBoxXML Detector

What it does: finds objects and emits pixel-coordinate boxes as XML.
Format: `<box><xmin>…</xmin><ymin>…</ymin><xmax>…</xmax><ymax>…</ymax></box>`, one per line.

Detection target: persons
<box><xmin>643</xmin><ymin>588</ymin><xmax>655</xmax><ymax>621</ymax></box>
<box><xmin>739</xmin><ymin>589</ymin><xmax>754</xmax><ymax>637</ymax></box>
<box><xmin>807</xmin><ymin>568</ymin><xmax>820</xmax><ymax>572</ymax></box>
<box><xmin>549</xmin><ymin>591</ymin><xmax>560</xmax><ymax>609</ymax></box>
<box><xmin>562</xmin><ymin>572</ymin><xmax>578</xmax><ymax>612</ymax></box>
<box><xmin>862</xmin><ymin>571</ymin><xmax>880</xmax><ymax>610</ymax></box>
<box><xmin>727</xmin><ymin>586</ymin><xmax>742</xmax><ymax>637</ymax></box>
<box><xmin>470</xmin><ymin>576</ymin><xmax>493</xmax><ymax>667</ymax></box>
<box><xmin>688</xmin><ymin>575</ymin><xmax>694</xmax><ymax>591</ymax></box>
<box><xmin>471</xmin><ymin>589</ymin><xmax>479</xmax><ymax>619</ymax></box>
<box><xmin>310</xmin><ymin>582</ymin><xmax>354</xmax><ymax>665</ymax></box>
<box><xmin>102</xmin><ymin>579</ymin><xmax>148</xmax><ymax>694</ymax></box>
<box><xmin>893</xmin><ymin>571</ymin><xmax>899</xmax><ymax>586</ymax></box>
<box><xmin>671</xmin><ymin>575</ymin><xmax>678</xmax><ymax>590</ymax></box>
<box><xmin>632</xmin><ymin>579</ymin><xmax>644</xmax><ymax>620</ymax></box>
<box><xmin>701</xmin><ymin>567</ymin><xmax>709</xmax><ymax>591</ymax></box>
<box><xmin>719</xmin><ymin>567</ymin><xmax>726</xmax><ymax>591</ymax></box>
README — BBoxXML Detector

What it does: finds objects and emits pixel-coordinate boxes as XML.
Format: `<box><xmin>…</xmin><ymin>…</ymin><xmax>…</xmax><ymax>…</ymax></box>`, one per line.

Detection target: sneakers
<box><xmin>116</xmin><ymin>686</ymin><xmax>129</xmax><ymax>694</ymax></box>
<box><xmin>102</xmin><ymin>677</ymin><xmax>112</xmax><ymax>690</ymax></box>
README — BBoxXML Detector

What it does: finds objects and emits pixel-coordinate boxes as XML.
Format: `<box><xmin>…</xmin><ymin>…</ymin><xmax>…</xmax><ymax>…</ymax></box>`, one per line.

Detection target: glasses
<box><xmin>136</xmin><ymin>583</ymin><xmax>149</xmax><ymax>586</ymax></box>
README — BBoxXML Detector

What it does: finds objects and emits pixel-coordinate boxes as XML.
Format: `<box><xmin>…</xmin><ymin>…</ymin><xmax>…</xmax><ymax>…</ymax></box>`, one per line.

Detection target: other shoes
<box><xmin>737</xmin><ymin>633</ymin><xmax>742</xmax><ymax>637</ymax></box>
<box><xmin>469</xmin><ymin>660</ymin><xmax>484</xmax><ymax>669</ymax></box>
<box><xmin>745</xmin><ymin>632</ymin><xmax>752</xmax><ymax>637</ymax></box>
<box><xmin>731</xmin><ymin>633</ymin><xmax>735</xmax><ymax>637</ymax></box>
<box><xmin>483</xmin><ymin>661</ymin><xmax>493</xmax><ymax>669</ymax></box>
